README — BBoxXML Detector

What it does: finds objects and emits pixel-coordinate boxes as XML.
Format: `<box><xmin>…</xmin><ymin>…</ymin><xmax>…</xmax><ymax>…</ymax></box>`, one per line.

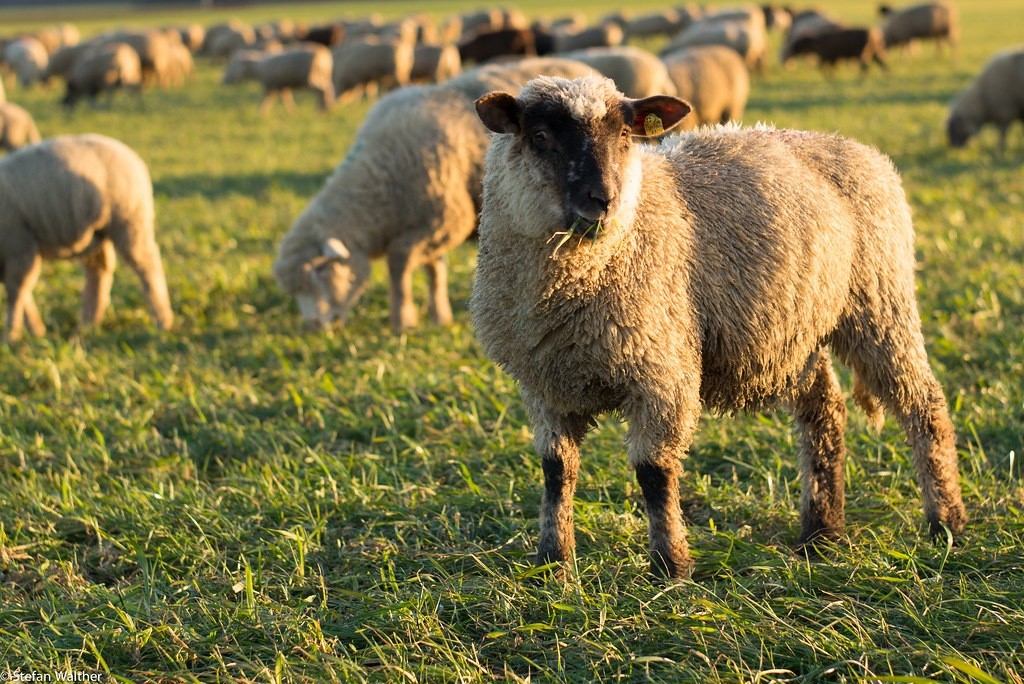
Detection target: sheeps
<box><xmin>0</xmin><ymin>0</ymin><xmax>1023</xmax><ymax>152</ymax></box>
<box><xmin>1</xmin><ymin>135</ymin><xmax>175</xmax><ymax>341</ymax></box>
<box><xmin>273</xmin><ymin>59</ymin><xmax>625</xmax><ymax>346</ymax></box>
<box><xmin>473</xmin><ymin>72</ymin><xmax>970</xmax><ymax>582</ymax></box>
